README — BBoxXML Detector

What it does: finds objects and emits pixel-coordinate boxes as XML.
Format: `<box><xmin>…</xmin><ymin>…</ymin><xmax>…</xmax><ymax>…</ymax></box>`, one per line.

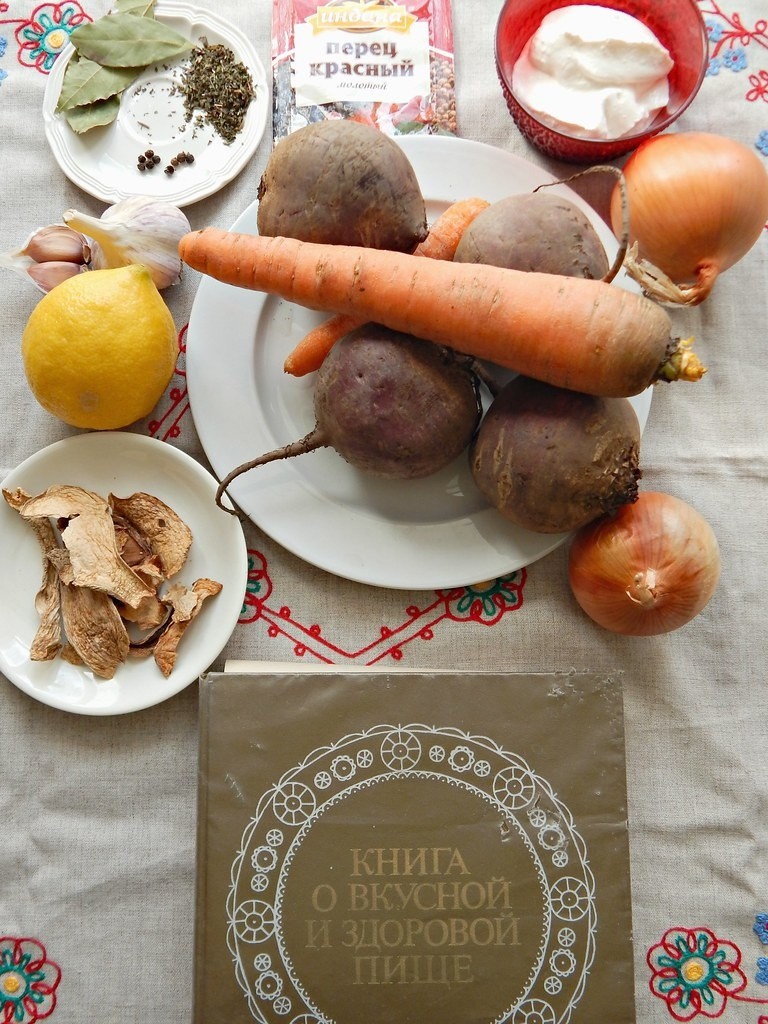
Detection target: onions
<box><xmin>611</xmin><ymin>132</ymin><xmax>768</xmax><ymax>308</ymax></box>
<box><xmin>568</xmin><ymin>492</ymin><xmax>721</xmax><ymax>637</ymax></box>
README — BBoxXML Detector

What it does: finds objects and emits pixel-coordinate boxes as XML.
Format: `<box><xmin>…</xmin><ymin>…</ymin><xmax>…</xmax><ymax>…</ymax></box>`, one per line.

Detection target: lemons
<box><xmin>22</xmin><ymin>263</ymin><xmax>177</xmax><ymax>431</ymax></box>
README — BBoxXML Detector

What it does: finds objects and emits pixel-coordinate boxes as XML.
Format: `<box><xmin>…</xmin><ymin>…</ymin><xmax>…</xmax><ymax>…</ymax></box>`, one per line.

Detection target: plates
<box><xmin>0</xmin><ymin>431</ymin><xmax>248</xmax><ymax>718</ymax></box>
<box><xmin>38</xmin><ymin>3</ymin><xmax>271</xmax><ymax>209</ymax></box>
<box><xmin>184</xmin><ymin>133</ymin><xmax>653</xmax><ymax>593</ymax></box>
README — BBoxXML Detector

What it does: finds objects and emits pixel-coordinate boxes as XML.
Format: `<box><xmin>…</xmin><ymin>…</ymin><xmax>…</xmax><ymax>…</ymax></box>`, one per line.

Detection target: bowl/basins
<box><xmin>492</xmin><ymin>0</ymin><xmax>712</xmax><ymax>165</ymax></box>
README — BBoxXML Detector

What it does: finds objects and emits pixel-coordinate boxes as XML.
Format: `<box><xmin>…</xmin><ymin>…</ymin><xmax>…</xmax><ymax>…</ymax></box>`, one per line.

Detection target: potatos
<box><xmin>216</xmin><ymin>327</ymin><xmax>641</xmax><ymax>534</ymax></box>
<box><xmin>256</xmin><ymin>120</ymin><xmax>630</xmax><ymax>284</ymax></box>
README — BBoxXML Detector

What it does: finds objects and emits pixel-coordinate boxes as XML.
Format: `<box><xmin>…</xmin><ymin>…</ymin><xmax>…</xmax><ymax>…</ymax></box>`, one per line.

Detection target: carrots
<box><xmin>177</xmin><ymin>197</ymin><xmax>708</xmax><ymax>399</ymax></box>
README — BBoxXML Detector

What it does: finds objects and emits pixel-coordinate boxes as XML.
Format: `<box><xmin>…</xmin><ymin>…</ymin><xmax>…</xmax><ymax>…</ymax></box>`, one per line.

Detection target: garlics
<box><xmin>2</xmin><ymin>195</ymin><xmax>192</xmax><ymax>297</ymax></box>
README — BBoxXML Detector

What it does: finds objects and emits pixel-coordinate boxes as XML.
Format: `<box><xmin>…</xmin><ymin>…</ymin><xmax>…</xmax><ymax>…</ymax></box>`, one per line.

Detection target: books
<box><xmin>190</xmin><ymin>662</ymin><xmax>637</xmax><ymax>1024</ymax></box>
